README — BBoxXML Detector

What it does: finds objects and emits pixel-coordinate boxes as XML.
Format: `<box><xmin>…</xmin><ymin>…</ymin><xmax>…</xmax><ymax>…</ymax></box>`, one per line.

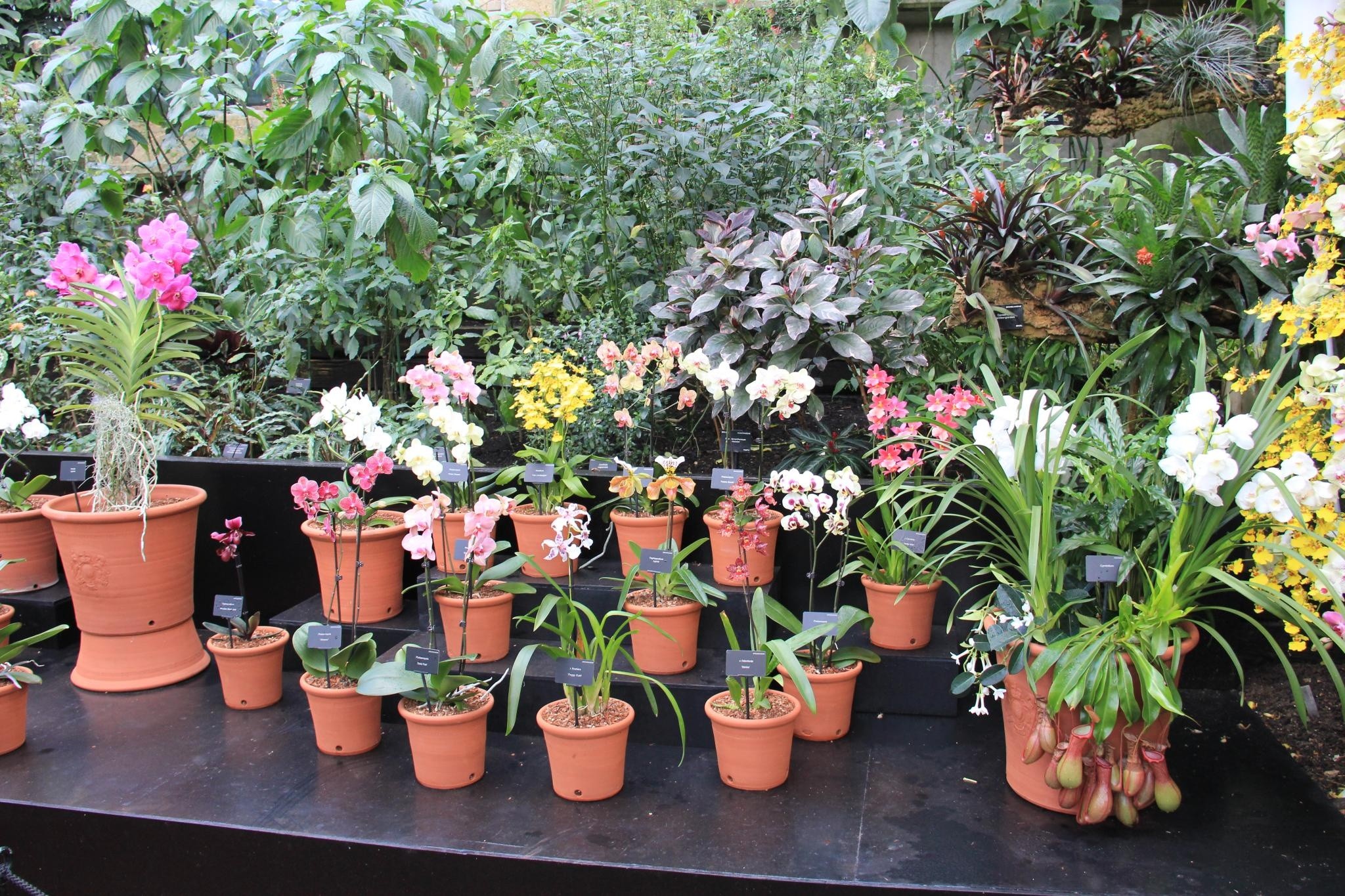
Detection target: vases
<box><xmin>623</xmin><ymin>589</ymin><xmax>704</xmax><ymax>675</ymax></box>
<box><xmin>206</xmin><ymin>626</ymin><xmax>290</xmax><ymax>709</ymax></box>
<box><xmin>0</xmin><ymin>604</ymin><xmax>15</xmax><ymax>647</ymax></box>
<box><xmin>42</xmin><ymin>484</ymin><xmax>212</xmax><ymax>693</ymax></box>
<box><xmin>298</xmin><ymin>661</ymin><xmax>381</xmax><ymax>755</ymax></box>
<box><xmin>536</xmin><ymin>696</ymin><xmax>635</xmax><ymax>802</ymax></box>
<box><xmin>508</xmin><ymin>502</ymin><xmax>587</xmax><ymax>578</ymax></box>
<box><xmin>610</xmin><ymin>505</ymin><xmax>688</xmax><ymax>580</ymax></box>
<box><xmin>0</xmin><ymin>495</ymin><xmax>59</xmax><ymax>594</ymax></box>
<box><xmin>0</xmin><ymin>0</ymin><xmax>1345</xmax><ymax>830</ymax></box>
<box><xmin>777</xmin><ymin>650</ymin><xmax>862</xmax><ymax>741</ymax></box>
<box><xmin>434</xmin><ymin>580</ymin><xmax>514</xmax><ymax>664</ymax></box>
<box><xmin>432</xmin><ymin>506</ymin><xmax>495</xmax><ymax>573</ymax></box>
<box><xmin>703</xmin><ymin>508</ymin><xmax>784</xmax><ymax>586</ymax></box>
<box><xmin>398</xmin><ymin>686</ymin><xmax>494</xmax><ymax>790</ymax></box>
<box><xmin>0</xmin><ymin>666</ymin><xmax>33</xmax><ymax>756</ymax></box>
<box><xmin>984</xmin><ymin>607</ymin><xmax>1200</xmax><ymax>816</ymax></box>
<box><xmin>704</xmin><ymin>688</ymin><xmax>801</xmax><ymax>792</ymax></box>
<box><xmin>861</xmin><ymin>569</ymin><xmax>942</xmax><ymax>650</ymax></box>
<box><xmin>301</xmin><ymin>510</ymin><xmax>408</xmax><ymax>625</ymax></box>
